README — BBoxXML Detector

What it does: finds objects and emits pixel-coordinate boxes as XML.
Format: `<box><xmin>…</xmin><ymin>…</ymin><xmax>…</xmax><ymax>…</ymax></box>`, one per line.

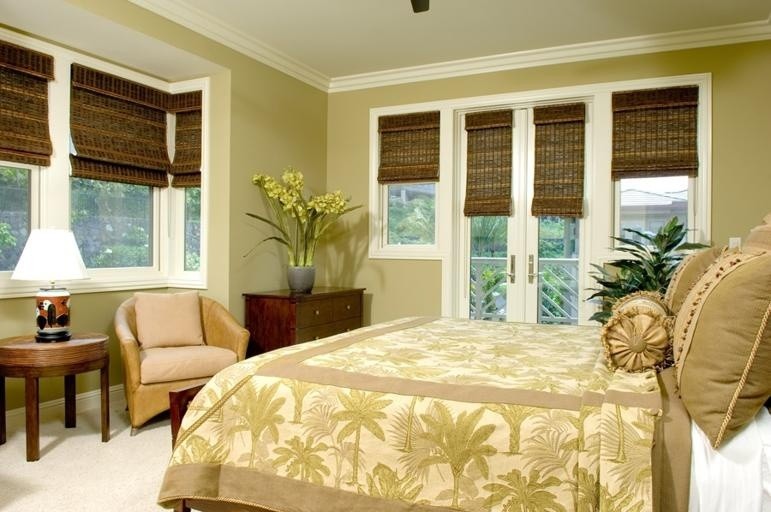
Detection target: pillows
<box><xmin>135</xmin><ymin>292</ymin><xmax>207</xmax><ymax>352</ymax></box>
<box><xmin>599</xmin><ymin>236</ymin><xmax>769</xmax><ymax>453</ymax></box>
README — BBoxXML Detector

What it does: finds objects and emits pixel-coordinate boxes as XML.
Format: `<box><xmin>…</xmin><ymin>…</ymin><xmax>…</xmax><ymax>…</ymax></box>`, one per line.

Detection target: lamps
<box><xmin>9</xmin><ymin>231</ymin><xmax>93</xmax><ymax>343</ymax></box>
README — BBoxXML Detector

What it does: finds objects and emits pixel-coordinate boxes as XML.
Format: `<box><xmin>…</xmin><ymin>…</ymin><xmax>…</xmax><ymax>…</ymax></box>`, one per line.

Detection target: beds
<box><xmin>156</xmin><ymin>207</ymin><xmax>770</xmax><ymax>512</ymax></box>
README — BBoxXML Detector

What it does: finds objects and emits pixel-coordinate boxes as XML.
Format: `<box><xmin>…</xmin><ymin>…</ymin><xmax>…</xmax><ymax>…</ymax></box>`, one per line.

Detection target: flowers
<box><xmin>240</xmin><ymin>163</ymin><xmax>366</xmax><ymax>266</ymax></box>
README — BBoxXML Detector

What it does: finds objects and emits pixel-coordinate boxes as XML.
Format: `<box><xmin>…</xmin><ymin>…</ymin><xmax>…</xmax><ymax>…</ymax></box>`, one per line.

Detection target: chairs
<box><xmin>115</xmin><ymin>291</ymin><xmax>252</xmax><ymax>438</ymax></box>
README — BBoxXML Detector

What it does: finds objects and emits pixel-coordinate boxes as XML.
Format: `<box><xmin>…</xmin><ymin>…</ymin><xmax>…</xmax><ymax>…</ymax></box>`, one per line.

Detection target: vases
<box><xmin>287</xmin><ymin>262</ymin><xmax>315</xmax><ymax>295</ymax></box>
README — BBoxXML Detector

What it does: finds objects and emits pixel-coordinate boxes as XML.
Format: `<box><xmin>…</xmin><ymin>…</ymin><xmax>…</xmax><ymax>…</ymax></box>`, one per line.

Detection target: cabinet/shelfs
<box><xmin>242</xmin><ymin>285</ymin><xmax>368</xmax><ymax>361</ymax></box>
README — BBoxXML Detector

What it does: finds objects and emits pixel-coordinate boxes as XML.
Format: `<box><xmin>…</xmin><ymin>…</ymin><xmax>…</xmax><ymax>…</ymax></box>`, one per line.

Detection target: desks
<box><xmin>1</xmin><ymin>332</ymin><xmax>111</xmax><ymax>463</ymax></box>
